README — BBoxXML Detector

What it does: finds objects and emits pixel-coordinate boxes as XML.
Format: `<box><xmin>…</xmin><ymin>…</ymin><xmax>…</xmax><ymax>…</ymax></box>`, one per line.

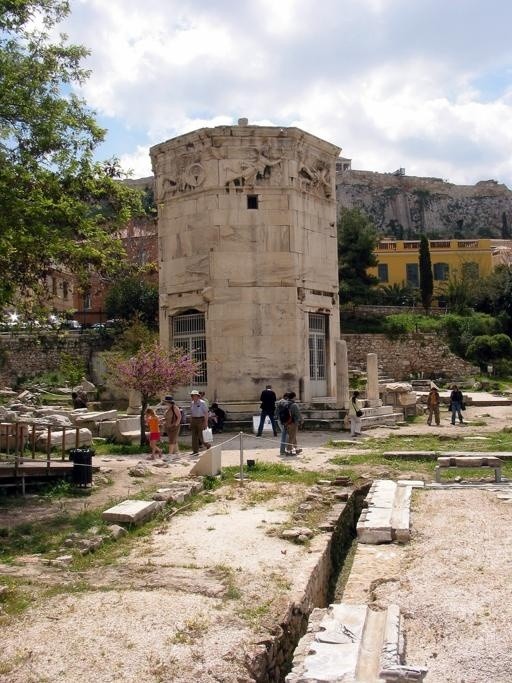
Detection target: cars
<box><xmin>0</xmin><ymin>314</ymin><xmax>122</xmax><ymax>332</ymax></box>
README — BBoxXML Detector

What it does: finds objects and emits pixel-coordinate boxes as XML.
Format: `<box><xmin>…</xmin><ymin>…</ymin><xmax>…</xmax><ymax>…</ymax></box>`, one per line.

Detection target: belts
<box><xmin>192</xmin><ymin>416</ymin><xmax>203</xmax><ymax>419</ymax></box>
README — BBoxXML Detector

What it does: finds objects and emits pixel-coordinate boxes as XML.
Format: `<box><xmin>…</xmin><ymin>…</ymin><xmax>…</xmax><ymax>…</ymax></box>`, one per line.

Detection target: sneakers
<box><xmin>257</xmin><ymin>433</ymin><xmax>276</xmax><ymax>438</ymax></box>
<box><xmin>162</xmin><ymin>454</ymin><xmax>180</xmax><ymax>461</ymax></box>
<box><xmin>352</xmin><ymin>431</ymin><xmax>361</xmax><ymax>436</ymax></box>
<box><xmin>280</xmin><ymin>449</ymin><xmax>302</xmax><ymax>456</ymax></box>
<box><xmin>450</xmin><ymin>422</ymin><xmax>462</xmax><ymax>425</ymax></box>
<box><xmin>147</xmin><ymin>450</ymin><xmax>162</xmax><ymax>460</ymax></box>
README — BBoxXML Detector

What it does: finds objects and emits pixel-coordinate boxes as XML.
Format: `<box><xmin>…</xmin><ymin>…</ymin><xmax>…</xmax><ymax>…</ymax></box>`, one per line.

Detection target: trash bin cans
<box><xmin>70</xmin><ymin>449</ymin><xmax>92</xmax><ymax>487</ymax></box>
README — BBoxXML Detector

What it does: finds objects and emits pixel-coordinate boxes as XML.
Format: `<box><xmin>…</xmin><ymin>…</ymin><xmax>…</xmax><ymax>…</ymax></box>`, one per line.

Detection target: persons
<box><xmin>426</xmin><ymin>386</ymin><xmax>440</xmax><ymax>425</ymax></box>
<box><xmin>77</xmin><ymin>388</ymin><xmax>88</xmax><ymax>404</ymax></box>
<box><xmin>71</xmin><ymin>391</ymin><xmax>88</xmax><ymax>409</ymax></box>
<box><xmin>162</xmin><ymin>394</ymin><xmax>183</xmax><ymax>462</ymax></box>
<box><xmin>255</xmin><ymin>384</ymin><xmax>278</xmax><ymax>437</ymax></box>
<box><xmin>223</xmin><ymin>144</ymin><xmax>286</xmax><ymax>185</ymax></box>
<box><xmin>348</xmin><ymin>390</ymin><xmax>363</xmax><ymax>437</ymax></box>
<box><xmin>284</xmin><ymin>391</ymin><xmax>303</xmax><ymax>456</ymax></box>
<box><xmin>449</xmin><ymin>384</ymin><xmax>463</xmax><ymax>425</ymax></box>
<box><xmin>145</xmin><ymin>407</ymin><xmax>163</xmax><ymax>459</ymax></box>
<box><xmin>275</xmin><ymin>391</ymin><xmax>290</xmax><ymax>456</ymax></box>
<box><xmin>187</xmin><ymin>388</ymin><xmax>226</xmax><ymax>455</ymax></box>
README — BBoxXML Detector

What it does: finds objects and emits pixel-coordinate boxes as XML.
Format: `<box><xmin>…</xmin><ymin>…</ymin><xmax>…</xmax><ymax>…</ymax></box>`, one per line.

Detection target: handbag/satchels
<box><xmin>202</xmin><ymin>427</ymin><xmax>213</xmax><ymax>444</ymax></box>
<box><xmin>356</xmin><ymin>410</ymin><xmax>363</xmax><ymax>417</ymax></box>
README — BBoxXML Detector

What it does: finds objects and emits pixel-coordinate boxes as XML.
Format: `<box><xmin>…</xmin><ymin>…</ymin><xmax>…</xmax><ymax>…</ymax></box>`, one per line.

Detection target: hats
<box><xmin>188</xmin><ymin>390</ymin><xmax>200</xmax><ymax>396</ymax></box>
<box><xmin>430</xmin><ymin>387</ymin><xmax>437</xmax><ymax>390</ymax></box>
<box><xmin>162</xmin><ymin>395</ymin><xmax>175</xmax><ymax>402</ymax></box>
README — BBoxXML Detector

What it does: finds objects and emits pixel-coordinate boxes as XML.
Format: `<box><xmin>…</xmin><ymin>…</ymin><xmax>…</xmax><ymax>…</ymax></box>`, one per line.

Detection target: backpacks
<box><xmin>278</xmin><ymin>402</ymin><xmax>295</xmax><ymax>426</ymax></box>
<box><xmin>172</xmin><ymin>405</ymin><xmax>187</xmax><ymax>424</ymax></box>
